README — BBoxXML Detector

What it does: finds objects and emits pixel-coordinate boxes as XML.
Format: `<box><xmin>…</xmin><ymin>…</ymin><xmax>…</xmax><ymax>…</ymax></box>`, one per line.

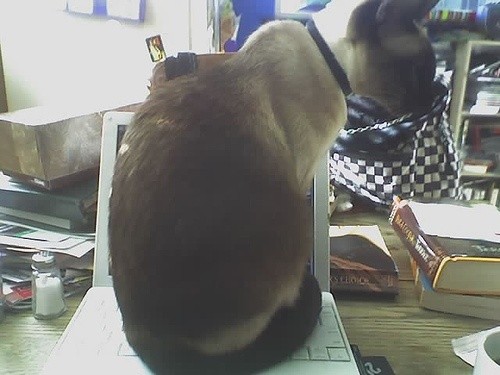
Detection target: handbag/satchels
<box><xmin>330</xmin><ymin>78</ymin><xmax>461</xmax><ymax>209</ymax></box>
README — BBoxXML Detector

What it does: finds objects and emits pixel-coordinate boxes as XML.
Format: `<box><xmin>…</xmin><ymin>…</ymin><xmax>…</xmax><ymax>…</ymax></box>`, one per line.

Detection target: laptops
<box><xmin>39</xmin><ymin>111</ymin><xmax>362</xmax><ymax>375</ymax></box>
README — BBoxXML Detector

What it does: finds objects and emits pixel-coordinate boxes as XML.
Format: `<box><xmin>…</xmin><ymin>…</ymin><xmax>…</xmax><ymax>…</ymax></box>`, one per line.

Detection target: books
<box><xmin>406</xmin><ymin>245</ymin><xmax>500</xmax><ymax>321</ymax></box>
<box><xmin>462</xmin><ymin>180</ymin><xmax>500</xmax><ymax>206</ymax></box>
<box><xmin>462</xmin><ymin>158</ymin><xmax>496</xmax><ymax>175</ymax></box>
<box><xmin>389</xmin><ymin>200</ymin><xmax>500</xmax><ymax>297</ymax></box>
<box><xmin>329</xmin><ymin>225</ymin><xmax>399</xmax><ymax>297</ymax></box>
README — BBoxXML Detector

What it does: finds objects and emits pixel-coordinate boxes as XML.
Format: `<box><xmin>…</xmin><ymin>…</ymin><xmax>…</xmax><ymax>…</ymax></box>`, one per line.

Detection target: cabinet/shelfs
<box><xmin>435</xmin><ymin>37</ymin><xmax>500</xmax><ymax>207</ymax></box>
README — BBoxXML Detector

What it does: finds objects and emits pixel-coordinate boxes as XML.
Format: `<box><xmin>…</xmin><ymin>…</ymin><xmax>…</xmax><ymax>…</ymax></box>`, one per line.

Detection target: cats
<box><xmin>106</xmin><ymin>0</ymin><xmax>437</xmax><ymax>375</ymax></box>
